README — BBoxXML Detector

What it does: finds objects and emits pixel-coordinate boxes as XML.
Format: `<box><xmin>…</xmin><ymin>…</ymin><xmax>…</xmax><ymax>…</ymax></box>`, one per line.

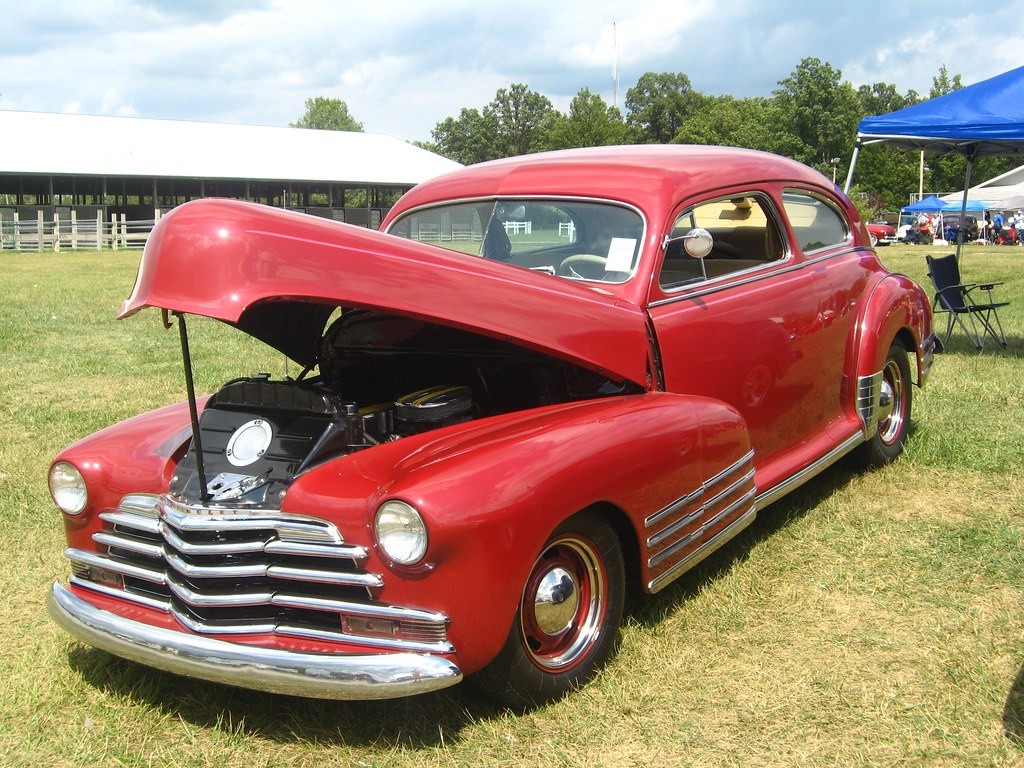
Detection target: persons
<box><xmin>908</xmin><ymin>210</ymin><xmax>1024</xmax><ymax>247</ymax></box>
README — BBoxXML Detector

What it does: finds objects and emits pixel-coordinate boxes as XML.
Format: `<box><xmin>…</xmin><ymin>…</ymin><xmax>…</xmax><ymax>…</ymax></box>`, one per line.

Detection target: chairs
<box><xmin>925</xmin><ymin>254</ymin><xmax>1011</xmax><ymax>356</ymax></box>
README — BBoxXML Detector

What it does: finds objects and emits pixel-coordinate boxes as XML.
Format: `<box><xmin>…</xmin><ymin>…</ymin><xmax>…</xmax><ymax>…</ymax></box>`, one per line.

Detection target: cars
<box><xmin>936</xmin><ymin>214</ymin><xmax>980</xmax><ymax>243</ymax></box>
<box><xmin>865</xmin><ymin>224</ymin><xmax>898</xmax><ymax>248</ymax></box>
<box><xmin>46</xmin><ymin>143</ymin><xmax>943</xmax><ymax>710</ymax></box>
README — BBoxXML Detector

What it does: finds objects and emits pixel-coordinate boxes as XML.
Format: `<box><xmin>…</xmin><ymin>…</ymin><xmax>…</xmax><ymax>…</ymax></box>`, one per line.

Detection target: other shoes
<box><xmin>1018</xmin><ymin>243</ymin><xmax>1023</xmax><ymax>246</ymax></box>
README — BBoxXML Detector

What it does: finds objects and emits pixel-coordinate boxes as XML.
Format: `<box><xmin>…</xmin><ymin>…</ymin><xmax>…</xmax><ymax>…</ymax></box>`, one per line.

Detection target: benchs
<box><xmin>660</xmin><ymin>258</ymin><xmax>771</xmax><ymax>279</ymax></box>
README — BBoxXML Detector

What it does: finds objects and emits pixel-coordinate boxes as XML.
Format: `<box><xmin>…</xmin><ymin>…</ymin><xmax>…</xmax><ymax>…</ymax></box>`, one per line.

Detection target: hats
<box><xmin>1018</xmin><ymin>210</ymin><xmax>1022</xmax><ymax>214</ymax></box>
<box><xmin>999</xmin><ymin>211</ymin><xmax>1004</xmax><ymax>214</ymax></box>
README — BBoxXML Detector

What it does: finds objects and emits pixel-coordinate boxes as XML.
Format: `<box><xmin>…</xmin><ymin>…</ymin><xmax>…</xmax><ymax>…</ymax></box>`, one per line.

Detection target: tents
<box><xmin>843</xmin><ymin>66</ymin><xmax>1024</xmax><ymax>194</ymax></box>
<box><xmin>942</xmin><ymin>199</ymin><xmax>991</xmax><ymax>246</ymax></box>
<box><xmin>897</xmin><ymin>195</ymin><xmax>948</xmax><ymax>241</ymax></box>
